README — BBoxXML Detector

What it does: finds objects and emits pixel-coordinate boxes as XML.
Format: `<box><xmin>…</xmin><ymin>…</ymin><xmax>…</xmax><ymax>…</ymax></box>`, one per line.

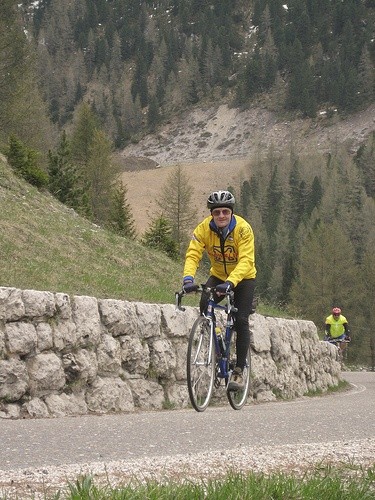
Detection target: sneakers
<box><xmin>228</xmin><ymin>368</ymin><xmax>245</xmax><ymax>391</ymax></box>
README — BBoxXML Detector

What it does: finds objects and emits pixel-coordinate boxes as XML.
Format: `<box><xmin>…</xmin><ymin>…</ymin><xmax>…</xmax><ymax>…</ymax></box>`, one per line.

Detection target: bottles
<box><xmin>215</xmin><ymin>326</ymin><xmax>226</xmax><ymax>353</ymax></box>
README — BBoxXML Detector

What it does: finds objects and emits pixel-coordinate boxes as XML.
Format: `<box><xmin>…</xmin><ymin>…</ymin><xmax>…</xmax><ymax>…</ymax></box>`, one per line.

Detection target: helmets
<box><xmin>207</xmin><ymin>190</ymin><xmax>236</xmax><ymax>207</ymax></box>
<box><xmin>332</xmin><ymin>307</ymin><xmax>342</xmax><ymax>314</ymax></box>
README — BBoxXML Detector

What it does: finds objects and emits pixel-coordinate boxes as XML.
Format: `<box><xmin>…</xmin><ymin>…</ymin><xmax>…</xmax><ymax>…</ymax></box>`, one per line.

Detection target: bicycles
<box><xmin>327</xmin><ymin>336</ymin><xmax>350</xmax><ymax>371</ymax></box>
<box><xmin>174</xmin><ymin>285</ymin><xmax>251</xmax><ymax>412</ymax></box>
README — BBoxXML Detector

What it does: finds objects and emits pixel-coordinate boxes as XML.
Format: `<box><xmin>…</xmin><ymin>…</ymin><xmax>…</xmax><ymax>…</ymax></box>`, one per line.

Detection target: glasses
<box><xmin>211</xmin><ymin>208</ymin><xmax>230</xmax><ymax>217</ymax></box>
<box><xmin>333</xmin><ymin>313</ymin><xmax>340</xmax><ymax>316</ymax></box>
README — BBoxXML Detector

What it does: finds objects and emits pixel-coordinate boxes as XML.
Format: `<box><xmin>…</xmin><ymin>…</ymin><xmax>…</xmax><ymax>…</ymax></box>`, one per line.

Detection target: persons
<box><xmin>182</xmin><ymin>190</ymin><xmax>257</xmax><ymax>391</ymax></box>
<box><xmin>324</xmin><ymin>307</ymin><xmax>351</xmax><ymax>352</ymax></box>
